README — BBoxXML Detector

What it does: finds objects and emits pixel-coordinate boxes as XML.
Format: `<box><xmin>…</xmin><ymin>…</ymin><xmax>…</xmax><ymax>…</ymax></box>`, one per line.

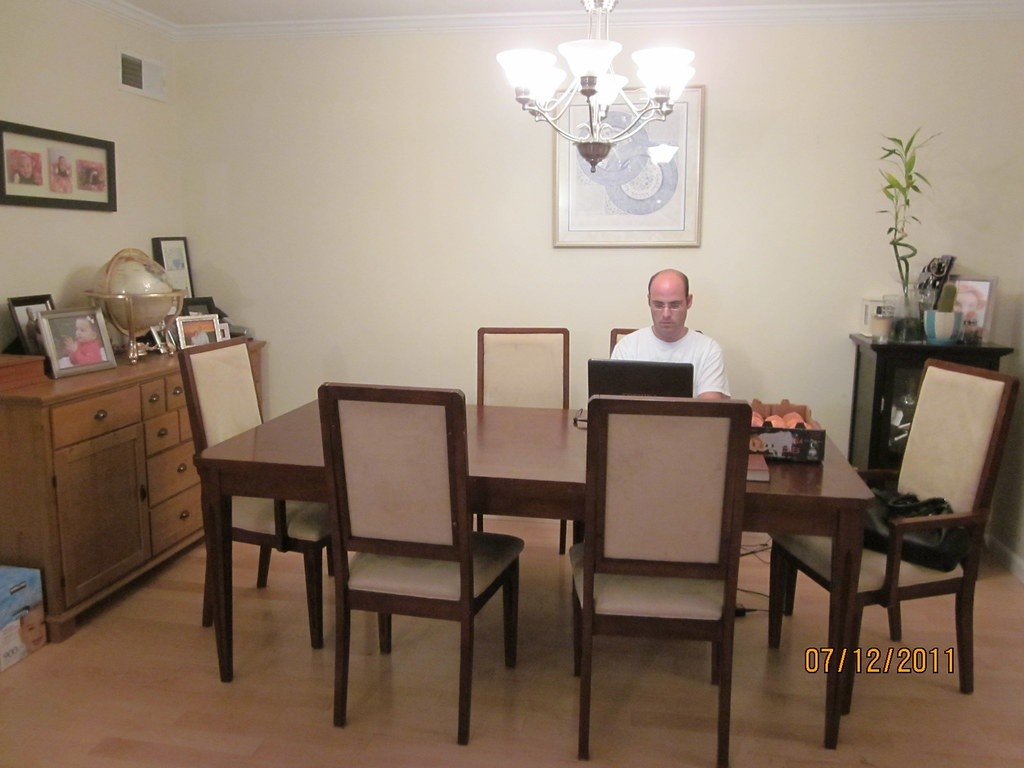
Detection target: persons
<box><xmin>612</xmin><ymin>270</ymin><xmax>731</xmax><ymax>399</ymax></box>
<box><xmin>191</xmin><ymin>327</ymin><xmax>209</xmax><ymax>345</ymax></box>
<box><xmin>84</xmin><ymin>162</ymin><xmax>99</xmax><ymax>184</ymax></box>
<box><xmin>55</xmin><ymin>156</ymin><xmax>70</xmax><ymax>176</ymax></box>
<box><xmin>65</xmin><ymin>314</ymin><xmax>102</xmax><ymax>365</ymax></box>
<box><xmin>13</xmin><ymin>154</ymin><xmax>35</xmax><ymax>184</ymax></box>
<box><xmin>27</xmin><ymin>306</ymin><xmax>38</xmax><ymax>347</ymax></box>
<box><xmin>957</xmin><ymin>284</ymin><xmax>986</xmax><ymax>332</ymax></box>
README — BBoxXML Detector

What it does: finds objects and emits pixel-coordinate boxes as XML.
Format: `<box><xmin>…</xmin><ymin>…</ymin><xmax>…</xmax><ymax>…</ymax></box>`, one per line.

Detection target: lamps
<box><xmin>495</xmin><ymin>0</ymin><xmax>697</xmax><ymax>178</ymax></box>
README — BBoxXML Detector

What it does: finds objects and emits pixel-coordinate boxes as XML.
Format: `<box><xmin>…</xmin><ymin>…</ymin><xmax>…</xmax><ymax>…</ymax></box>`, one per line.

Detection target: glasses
<box><xmin>573</xmin><ymin>408</ymin><xmax>587</xmax><ymax>427</ymax></box>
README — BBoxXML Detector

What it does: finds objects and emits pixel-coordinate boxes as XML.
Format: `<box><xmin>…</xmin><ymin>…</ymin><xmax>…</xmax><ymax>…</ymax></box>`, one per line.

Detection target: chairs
<box><xmin>177</xmin><ymin>328</ymin><xmax>1015</xmax><ymax>768</ymax></box>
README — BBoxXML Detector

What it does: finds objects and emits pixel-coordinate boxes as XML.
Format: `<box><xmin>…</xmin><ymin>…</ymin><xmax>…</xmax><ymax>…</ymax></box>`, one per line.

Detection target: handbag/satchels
<box><xmin>858</xmin><ymin>489</ymin><xmax>970</xmax><ymax>573</ymax></box>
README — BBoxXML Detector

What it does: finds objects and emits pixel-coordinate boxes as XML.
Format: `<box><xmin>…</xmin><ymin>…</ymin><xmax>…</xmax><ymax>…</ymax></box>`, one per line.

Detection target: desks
<box><xmin>200</xmin><ymin>401</ymin><xmax>876</xmax><ymax>751</ymax></box>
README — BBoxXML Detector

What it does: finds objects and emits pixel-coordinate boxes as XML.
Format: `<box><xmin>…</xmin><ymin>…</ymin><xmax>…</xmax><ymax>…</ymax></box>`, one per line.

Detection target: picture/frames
<box><xmin>952</xmin><ymin>272</ymin><xmax>1001</xmax><ymax>347</ymax></box>
<box><xmin>0</xmin><ymin>115</ymin><xmax>117</xmax><ymax>210</ymax></box>
<box><xmin>551</xmin><ymin>90</ymin><xmax>706</xmax><ymax>247</ymax></box>
<box><xmin>5</xmin><ymin>236</ymin><xmax>253</xmax><ymax>389</ymax></box>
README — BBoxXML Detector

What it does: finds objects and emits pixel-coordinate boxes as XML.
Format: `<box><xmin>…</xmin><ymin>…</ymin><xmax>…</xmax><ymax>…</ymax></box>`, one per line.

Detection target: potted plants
<box><xmin>921</xmin><ymin>283</ymin><xmax>965</xmax><ymax>347</ymax></box>
<box><xmin>876</xmin><ymin>127</ymin><xmax>942</xmax><ymax>344</ymax></box>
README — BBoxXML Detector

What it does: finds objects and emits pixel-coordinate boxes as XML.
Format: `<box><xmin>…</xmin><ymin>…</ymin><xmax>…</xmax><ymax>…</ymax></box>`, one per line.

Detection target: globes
<box><xmin>79</xmin><ymin>248</ymin><xmax>184</xmax><ymax>365</ymax></box>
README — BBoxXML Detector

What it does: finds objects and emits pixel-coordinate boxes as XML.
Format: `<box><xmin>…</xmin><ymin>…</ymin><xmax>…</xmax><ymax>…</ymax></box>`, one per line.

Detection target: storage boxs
<box><xmin>748</xmin><ymin>399</ymin><xmax>826</xmax><ymax>463</ymax></box>
<box><xmin>0</xmin><ymin>563</ymin><xmax>48</xmax><ymax>672</ymax></box>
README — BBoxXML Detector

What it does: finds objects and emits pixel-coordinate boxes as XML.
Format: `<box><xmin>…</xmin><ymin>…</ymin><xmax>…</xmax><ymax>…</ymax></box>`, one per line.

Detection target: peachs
<box><xmin>751</xmin><ymin>412</ymin><xmax>813</xmax><ymax>429</ymax></box>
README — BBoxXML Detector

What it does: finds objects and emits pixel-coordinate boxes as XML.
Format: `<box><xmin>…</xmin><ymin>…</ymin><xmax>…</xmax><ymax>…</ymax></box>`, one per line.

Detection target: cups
<box><xmin>872</xmin><ymin>316</ymin><xmax>893</xmax><ymax>344</ymax></box>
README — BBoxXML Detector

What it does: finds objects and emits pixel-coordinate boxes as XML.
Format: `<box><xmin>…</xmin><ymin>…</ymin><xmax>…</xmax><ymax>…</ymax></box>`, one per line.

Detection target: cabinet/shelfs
<box><xmin>845</xmin><ymin>330</ymin><xmax>1015</xmax><ymax>561</ymax></box>
<box><xmin>0</xmin><ymin>340</ymin><xmax>273</xmax><ymax>644</ymax></box>
